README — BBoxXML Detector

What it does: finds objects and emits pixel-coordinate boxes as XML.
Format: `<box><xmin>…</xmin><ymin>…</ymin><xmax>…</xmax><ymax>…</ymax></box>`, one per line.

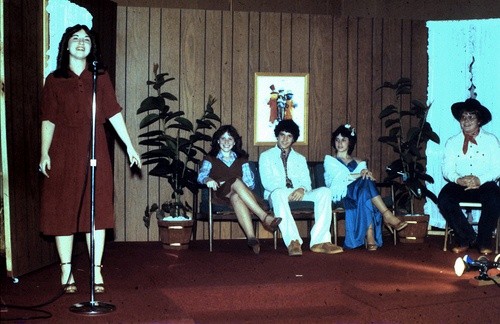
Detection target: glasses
<box><xmin>460</xmin><ymin>116</ymin><xmax>478</xmax><ymax>121</ymax></box>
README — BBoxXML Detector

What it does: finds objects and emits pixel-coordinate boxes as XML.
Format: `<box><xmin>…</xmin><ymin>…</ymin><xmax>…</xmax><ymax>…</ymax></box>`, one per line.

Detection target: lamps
<box><xmin>454</xmin><ymin>254</ymin><xmax>500</xmax><ymax>281</ymax></box>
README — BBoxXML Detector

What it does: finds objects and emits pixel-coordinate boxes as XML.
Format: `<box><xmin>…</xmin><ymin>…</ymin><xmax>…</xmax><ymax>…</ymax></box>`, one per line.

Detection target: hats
<box><xmin>451</xmin><ymin>98</ymin><xmax>492</xmax><ymax>126</ymax></box>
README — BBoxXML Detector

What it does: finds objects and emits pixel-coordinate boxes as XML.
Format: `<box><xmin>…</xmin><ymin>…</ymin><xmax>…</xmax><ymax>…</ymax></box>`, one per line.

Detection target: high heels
<box><xmin>60</xmin><ymin>262</ymin><xmax>77</xmax><ymax>293</ymax></box>
<box><xmin>249</xmin><ymin>239</ymin><xmax>261</xmax><ymax>254</ymax></box>
<box><xmin>263</xmin><ymin>215</ymin><xmax>282</xmax><ymax>231</ymax></box>
<box><xmin>365</xmin><ymin>227</ymin><xmax>378</xmax><ymax>251</ymax></box>
<box><xmin>382</xmin><ymin>209</ymin><xmax>408</xmax><ymax>234</ymax></box>
<box><xmin>93</xmin><ymin>264</ymin><xmax>103</xmax><ymax>293</ymax></box>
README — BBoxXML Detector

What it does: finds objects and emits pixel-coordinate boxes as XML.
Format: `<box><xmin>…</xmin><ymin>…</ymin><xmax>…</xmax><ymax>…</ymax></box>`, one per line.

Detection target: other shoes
<box><xmin>480</xmin><ymin>248</ymin><xmax>492</xmax><ymax>254</ymax></box>
<box><xmin>452</xmin><ymin>245</ymin><xmax>468</xmax><ymax>253</ymax></box>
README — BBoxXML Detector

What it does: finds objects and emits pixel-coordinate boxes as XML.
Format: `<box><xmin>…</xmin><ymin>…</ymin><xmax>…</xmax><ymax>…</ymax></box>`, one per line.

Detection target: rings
<box><xmin>39</xmin><ymin>169</ymin><xmax>41</xmax><ymax>171</ymax></box>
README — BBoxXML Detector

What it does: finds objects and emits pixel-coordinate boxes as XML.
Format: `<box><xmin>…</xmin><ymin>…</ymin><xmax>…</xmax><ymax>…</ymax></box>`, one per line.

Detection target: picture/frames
<box><xmin>251</xmin><ymin>72</ymin><xmax>309</xmax><ymax>142</ymax></box>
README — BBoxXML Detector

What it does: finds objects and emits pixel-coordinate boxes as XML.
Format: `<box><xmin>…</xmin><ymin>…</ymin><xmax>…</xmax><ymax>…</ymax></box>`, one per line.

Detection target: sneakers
<box><xmin>288</xmin><ymin>241</ymin><xmax>302</xmax><ymax>255</ymax></box>
<box><xmin>311</xmin><ymin>243</ymin><xmax>344</xmax><ymax>254</ymax></box>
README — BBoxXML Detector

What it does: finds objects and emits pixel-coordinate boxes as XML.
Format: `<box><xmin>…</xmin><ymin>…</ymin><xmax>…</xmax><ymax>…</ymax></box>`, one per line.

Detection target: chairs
<box><xmin>192</xmin><ymin>160</ymin><xmax>397</xmax><ymax>250</ymax></box>
<box><xmin>444</xmin><ymin>179</ymin><xmax>500</xmax><ymax>254</ymax></box>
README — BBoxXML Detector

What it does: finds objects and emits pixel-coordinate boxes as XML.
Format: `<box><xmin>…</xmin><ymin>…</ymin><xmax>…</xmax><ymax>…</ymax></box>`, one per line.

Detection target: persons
<box><xmin>197</xmin><ymin>125</ymin><xmax>282</xmax><ymax>255</ymax></box>
<box><xmin>259</xmin><ymin>119</ymin><xmax>343</xmax><ymax>256</ymax></box>
<box><xmin>436</xmin><ymin>99</ymin><xmax>500</xmax><ymax>255</ymax></box>
<box><xmin>39</xmin><ymin>24</ymin><xmax>141</xmax><ymax>294</ymax></box>
<box><xmin>323</xmin><ymin>124</ymin><xmax>409</xmax><ymax>252</ymax></box>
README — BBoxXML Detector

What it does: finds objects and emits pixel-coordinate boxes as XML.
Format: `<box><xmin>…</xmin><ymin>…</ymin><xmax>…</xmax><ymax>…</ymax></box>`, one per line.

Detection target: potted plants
<box><xmin>136</xmin><ymin>62</ymin><xmax>222</xmax><ymax>251</ymax></box>
<box><xmin>376</xmin><ymin>77</ymin><xmax>440</xmax><ymax>244</ymax></box>
